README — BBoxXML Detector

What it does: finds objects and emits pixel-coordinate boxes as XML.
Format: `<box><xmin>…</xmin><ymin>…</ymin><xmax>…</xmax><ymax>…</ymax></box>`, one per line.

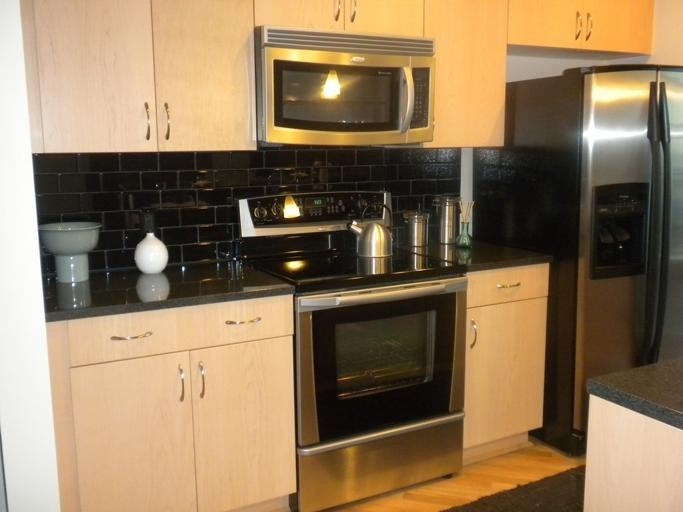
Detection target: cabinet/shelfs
<box><xmin>509</xmin><ymin>1</ymin><xmax>657</xmax><ymax>57</ymax></box>
<box><xmin>461</xmin><ymin>262</ymin><xmax>551</xmax><ymax>467</ymax></box>
<box><xmin>20</xmin><ymin>0</ymin><xmax>254</xmax><ymax>153</ymax></box>
<box><xmin>253</xmin><ymin>0</ymin><xmax>508</xmax><ymax>150</ymax></box>
<box><xmin>45</xmin><ymin>286</ymin><xmax>299</xmax><ymax>512</ymax></box>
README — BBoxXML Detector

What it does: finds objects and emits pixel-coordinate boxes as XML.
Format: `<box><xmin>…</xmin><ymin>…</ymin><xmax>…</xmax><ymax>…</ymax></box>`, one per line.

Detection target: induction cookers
<box><xmin>239</xmin><ymin>230</ymin><xmax>467</xmax><ymax>291</ymax></box>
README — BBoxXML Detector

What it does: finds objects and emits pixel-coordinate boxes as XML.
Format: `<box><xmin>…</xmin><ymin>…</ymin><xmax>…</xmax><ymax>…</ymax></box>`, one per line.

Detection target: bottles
<box><xmin>456</xmin><ymin>221</ymin><xmax>473</xmax><ymax>246</ymax></box>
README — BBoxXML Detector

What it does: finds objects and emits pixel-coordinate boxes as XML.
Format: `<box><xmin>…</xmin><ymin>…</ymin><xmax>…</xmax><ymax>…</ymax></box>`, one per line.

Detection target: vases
<box><xmin>133</xmin><ymin>232</ymin><xmax>169</xmax><ymax>274</ymax></box>
<box><xmin>136</xmin><ymin>274</ymin><xmax>170</xmax><ymax>302</ymax></box>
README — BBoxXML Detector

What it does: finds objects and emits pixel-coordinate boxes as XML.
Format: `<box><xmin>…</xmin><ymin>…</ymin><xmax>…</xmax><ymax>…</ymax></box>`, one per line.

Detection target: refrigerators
<box><xmin>499</xmin><ymin>63</ymin><xmax>681</xmax><ymax>458</ymax></box>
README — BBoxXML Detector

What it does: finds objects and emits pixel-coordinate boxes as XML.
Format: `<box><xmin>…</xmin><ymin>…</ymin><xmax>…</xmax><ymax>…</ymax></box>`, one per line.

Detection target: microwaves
<box><xmin>255</xmin><ymin>25</ymin><xmax>437</xmax><ymax>147</ymax></box>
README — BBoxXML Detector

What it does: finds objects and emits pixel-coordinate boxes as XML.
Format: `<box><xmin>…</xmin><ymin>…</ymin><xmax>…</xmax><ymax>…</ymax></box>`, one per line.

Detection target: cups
<box><xmin>403</xmin><ymin>211</ymin><xmax>430</xmax><ymax>245</ymax></box>
<box><xmin>433</xmin><ymin>196</ymin><xmax>463</xmax><ymax>243</ymax></box>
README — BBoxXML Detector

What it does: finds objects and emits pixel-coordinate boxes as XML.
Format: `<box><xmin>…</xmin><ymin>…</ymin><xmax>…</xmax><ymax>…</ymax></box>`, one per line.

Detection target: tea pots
<box><xmin>345</xmin><ymin>201</ymin><xmax>395</xmax><ymax>258</ymax></box>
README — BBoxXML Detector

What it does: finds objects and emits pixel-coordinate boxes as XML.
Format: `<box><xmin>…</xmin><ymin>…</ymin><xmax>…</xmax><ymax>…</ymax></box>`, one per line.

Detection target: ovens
<box><xmin>294</xmin><ymin>275</ymin><xmax>470</xmax><ymax>447</ymax></box>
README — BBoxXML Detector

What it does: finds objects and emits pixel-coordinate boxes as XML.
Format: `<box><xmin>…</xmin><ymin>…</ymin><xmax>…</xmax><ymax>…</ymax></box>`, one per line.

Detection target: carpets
<box><xmin>440</xmin><ymin>464</ymin><xmax>583</xmax><ymax>512</ymax></box>
